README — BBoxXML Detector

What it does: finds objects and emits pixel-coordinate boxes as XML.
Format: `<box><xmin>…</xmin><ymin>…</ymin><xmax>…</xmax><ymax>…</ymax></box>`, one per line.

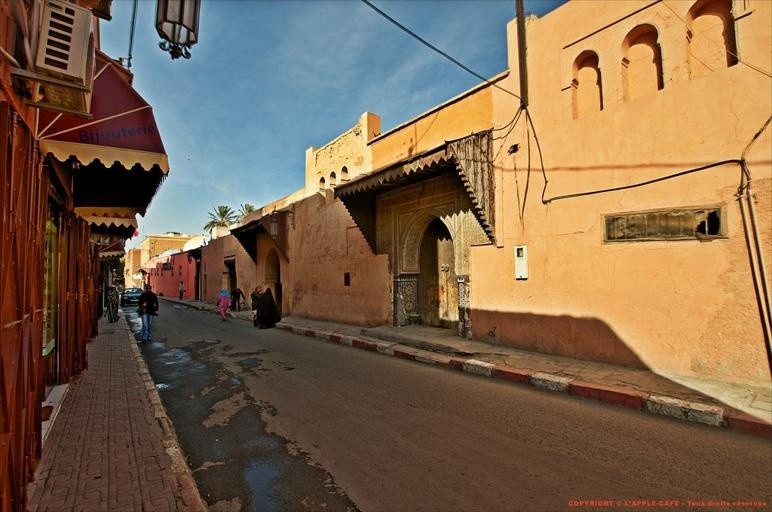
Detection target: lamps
<box><xmin>154</xmin><ymin>0</ymin><xmax>201</xmax><ymax>59</ymax></box>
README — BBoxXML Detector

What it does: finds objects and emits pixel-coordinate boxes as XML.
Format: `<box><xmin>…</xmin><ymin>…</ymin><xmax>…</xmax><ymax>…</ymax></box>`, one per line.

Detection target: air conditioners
<box><xmin>34</xmin><ymin>0</ymin><xmax>96</xmax><ymax>115</ymax></box>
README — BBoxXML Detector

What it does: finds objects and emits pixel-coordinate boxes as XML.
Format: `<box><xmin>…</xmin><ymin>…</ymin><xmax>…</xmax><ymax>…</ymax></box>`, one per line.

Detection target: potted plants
<box><xmin>201</xmin><ymin>205</ymin><xmax>239</xmax><ymax>238</ymax></box>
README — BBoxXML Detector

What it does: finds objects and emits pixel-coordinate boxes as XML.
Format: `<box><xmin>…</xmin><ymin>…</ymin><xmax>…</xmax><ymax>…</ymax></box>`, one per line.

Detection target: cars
<box><xmin>120</xmin><ymin>287</ymin><xmax>144</xmax><ymax>307</ymax></box>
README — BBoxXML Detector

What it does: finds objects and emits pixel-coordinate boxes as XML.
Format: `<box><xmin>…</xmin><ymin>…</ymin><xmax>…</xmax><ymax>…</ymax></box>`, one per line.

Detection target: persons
<box><xmin>251</xmin><ymin>285</ymin><xmax>278</xmax><ymax>329</ymax></box>
<box><xmin>231</xmin><ymin>287</ymin><xmax>245</xmax><ymax>312</ymax></box>
<box><xmin>179</xmin><ymin>281</ymin><xmax>186</xmax><ymax>300</ymax></box>
<box><xmin>216</xmin><ymin>289</ymin><xmax>232</xmax><ymax>321</ymax></box>
<box><xmin>137</xmin><ymin>285</ymin><xmax>159</xmax><ymax>343</ymax></box>
